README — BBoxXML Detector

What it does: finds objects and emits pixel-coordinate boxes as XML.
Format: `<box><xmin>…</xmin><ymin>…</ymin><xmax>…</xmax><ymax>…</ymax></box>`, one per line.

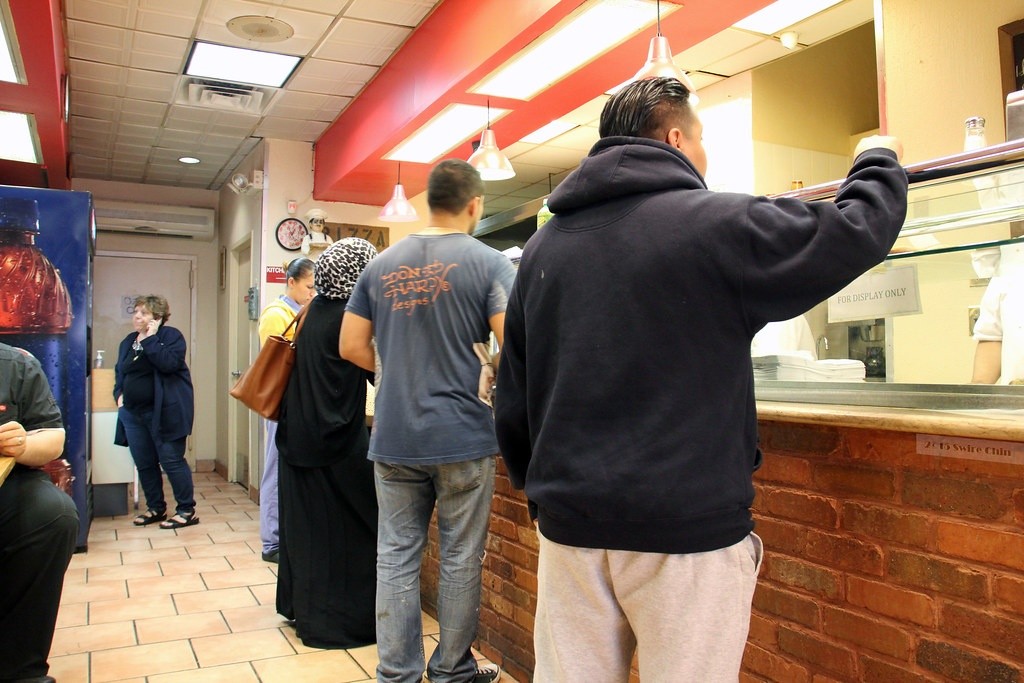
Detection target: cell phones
<box><xmin>155</xmin><ymin>314</ymin><xmax>161</xmax><ymax>321</ymax></box>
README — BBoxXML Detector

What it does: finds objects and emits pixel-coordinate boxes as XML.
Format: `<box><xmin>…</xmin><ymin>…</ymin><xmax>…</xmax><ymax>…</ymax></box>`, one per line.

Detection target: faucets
<box><xmin>816</xmin><ymin>335</ymin><xmax>829</xmax><ymax>360</ymax></box>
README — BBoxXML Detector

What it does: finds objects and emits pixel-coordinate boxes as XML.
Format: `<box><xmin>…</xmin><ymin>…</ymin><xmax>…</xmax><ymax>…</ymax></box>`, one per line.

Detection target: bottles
<box><xmin>537</xmin><ymin>199</ymin><xmax>555</xmax><ymax>230</ymax></box>
<box><xmin>964</xmin><ymin>117</ymin><xmax>987</xmax><ymax>152</ymax></box>
<box><xmin>0</xmin><ymin>199</ymin><xmax>72</xmax><ymax>499</ymax></box>
<box><xmin>792</xmin><ymin>181</ymin><xmax>803</xmax><ymax>191</ymax></box>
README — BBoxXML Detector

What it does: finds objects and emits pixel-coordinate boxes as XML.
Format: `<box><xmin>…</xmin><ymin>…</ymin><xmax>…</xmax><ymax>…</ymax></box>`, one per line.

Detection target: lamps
<box><xmin>467</xmin><ymin>100</ymin><xmax>516</xmax><ymax>180</ymax></box>
<box><xmin>632</xmin><ymin>0</ymin><xmax>700</xmax><ymax>104</ymax></box>
<box><xmin>226</xmin><ymin>170</ymin><xmax>263</xmax><ymax>196</ymax></box>
<box><xmin>377</xmin><ymin>161</ymin><xmax>419</xmax><ymax>222</ymax></box>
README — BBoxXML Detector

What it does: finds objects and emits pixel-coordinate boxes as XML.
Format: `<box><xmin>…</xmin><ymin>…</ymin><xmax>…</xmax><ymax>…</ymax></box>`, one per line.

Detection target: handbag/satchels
<box><xmin>228</xmin><ymin>297</ymin><xmax>314</xmax><ymax>422</ymax></box>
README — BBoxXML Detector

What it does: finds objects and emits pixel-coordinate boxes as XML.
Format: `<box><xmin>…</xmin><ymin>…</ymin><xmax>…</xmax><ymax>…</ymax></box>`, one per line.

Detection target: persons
<box><xmin>751</xmin><ymin>315</ymin><xmax>818</xmax><ymax>360</ymax></box>
<box><xmin>0</xmin><ymin>344</ymin><xmax>80</xmax><ymax>683</ymax></box>
<box><xmin>340</xmin><ymin>159</ymin><xmax>515</xmax><ymax>683</ymax></box>
<box><xmin>112</xmin><ymin>294</ymin><xmax>200</xmax><ymax>529</ymax></box>
<box><xmin>493</xmin><ymin>75</ymin><xmax>906</xmax><ymax>683</ymax></box>
<box><xmin>258</xmin><ymin>256</ymin><xmax>316</xmax><ymax>563</ymax></box>
<box><xmin>970</xmin><ymin>235</ymin><xmax>1024</xmax><ymax>386</ymax></box>
<box><xmin>275</xmin><ymin>237</ymin><xmax>382</xmax><ymax>650</ymax></box>
<box><xmin>301</xmin><ymin>209</ymin><xmax>333</xmax><ymax>263</ymax></box>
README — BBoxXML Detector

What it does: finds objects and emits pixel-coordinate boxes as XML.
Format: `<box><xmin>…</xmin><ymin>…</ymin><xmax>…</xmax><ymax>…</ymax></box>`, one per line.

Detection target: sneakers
<box><xmin>471</xmin><ymin>664</ymin><xmax>501</xmax><ymax>683</ymax></box>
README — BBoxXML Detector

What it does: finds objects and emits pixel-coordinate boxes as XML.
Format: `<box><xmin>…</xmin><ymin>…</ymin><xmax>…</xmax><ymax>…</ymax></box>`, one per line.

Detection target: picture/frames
<box><xmin>219</xmin><ymin>248</ymin><xmax>225</xmax><ymax>289</ymax></box>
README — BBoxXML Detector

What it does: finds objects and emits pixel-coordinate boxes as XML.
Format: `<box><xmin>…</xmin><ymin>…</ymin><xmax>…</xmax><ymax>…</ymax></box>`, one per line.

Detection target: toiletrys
<box><xmin>93</xmin><ymin>349</ymin><xmax>107</xmax><ymax>368</ymax></box>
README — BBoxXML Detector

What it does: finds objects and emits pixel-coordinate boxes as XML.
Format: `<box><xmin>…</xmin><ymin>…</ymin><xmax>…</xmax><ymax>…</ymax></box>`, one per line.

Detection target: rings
<box><xmin>18</xmin><ymin>437</ymin><xmax>21</xmax><ymax>444</ymax></box>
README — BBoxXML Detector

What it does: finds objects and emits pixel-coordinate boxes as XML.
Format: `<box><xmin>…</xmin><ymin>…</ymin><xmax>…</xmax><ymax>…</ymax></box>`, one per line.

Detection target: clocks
<box><xmin>276</xmin><ymin>218</ymin><xmax>308</xmax><ymax>251</ymax></box>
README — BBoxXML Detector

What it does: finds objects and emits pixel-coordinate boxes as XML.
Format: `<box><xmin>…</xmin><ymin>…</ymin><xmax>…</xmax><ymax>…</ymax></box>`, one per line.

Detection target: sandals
<box><xmin>134</xmin><ymin>507</ymin><xmax>167</xmax><ymax>526</ymax></box>
<box><xmin>160</xmin><ymin>510</ymin><xmax>200</xmax><ymax>529</ymax></box>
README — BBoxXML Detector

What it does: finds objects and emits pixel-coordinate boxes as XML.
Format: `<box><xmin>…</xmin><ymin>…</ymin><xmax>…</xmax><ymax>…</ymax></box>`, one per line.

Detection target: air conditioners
<box><xmin>93</xmin><ymin>199</ymin><xmax>214</xmax><ymax>242</ymax></box>
